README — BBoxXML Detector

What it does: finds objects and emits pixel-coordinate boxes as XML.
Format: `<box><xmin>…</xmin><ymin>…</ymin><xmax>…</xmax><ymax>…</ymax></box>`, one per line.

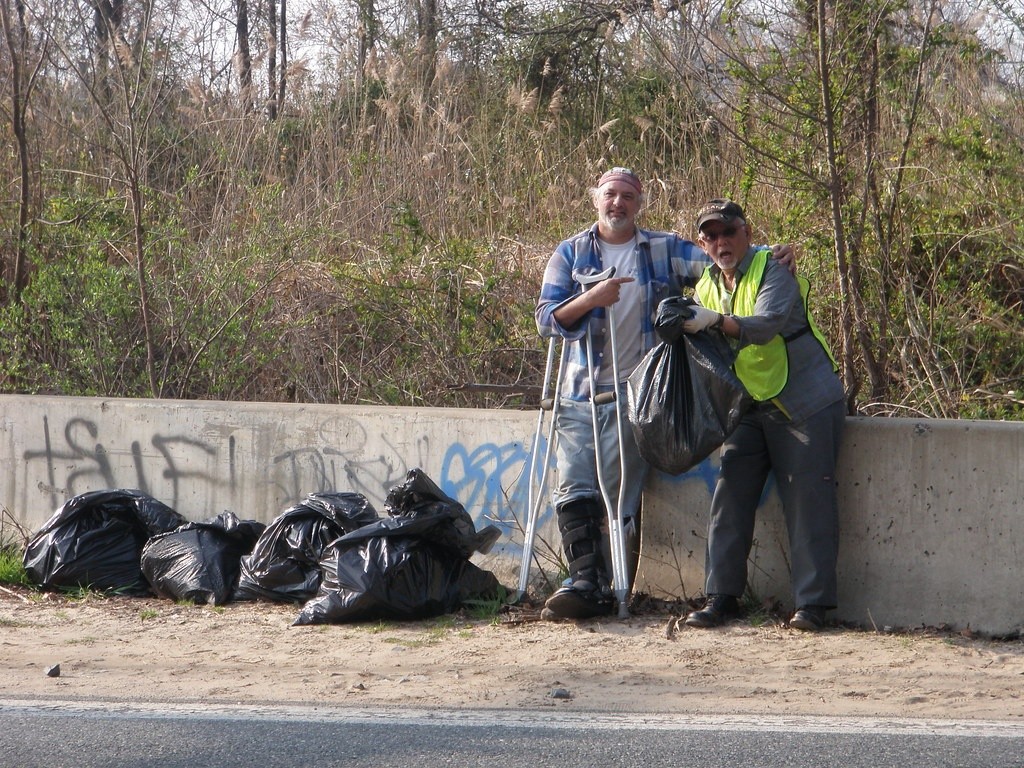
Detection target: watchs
<box><xmin>712</xmin><ymin>314</ymin><xmax>725</xmax><ymax>330</ymax></box>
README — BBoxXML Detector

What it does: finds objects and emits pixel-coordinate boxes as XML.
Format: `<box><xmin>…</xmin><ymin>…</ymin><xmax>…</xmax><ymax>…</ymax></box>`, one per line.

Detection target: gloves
<box><xmin>684</xmin><ymin>305</ymin><xmax>718</xmax><ymax>333</ymax></box>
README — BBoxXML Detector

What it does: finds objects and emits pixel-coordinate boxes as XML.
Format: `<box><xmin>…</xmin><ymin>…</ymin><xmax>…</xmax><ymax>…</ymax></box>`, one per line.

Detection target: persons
<box><xmin>682</xmin><ymin>198</ymin><xmax>848</xmax><ymax>630</ymax></box>
<box><xmin>534</xmin><ymin>167</ymin><xmax>798</xmax><ymax>621</ymax></box>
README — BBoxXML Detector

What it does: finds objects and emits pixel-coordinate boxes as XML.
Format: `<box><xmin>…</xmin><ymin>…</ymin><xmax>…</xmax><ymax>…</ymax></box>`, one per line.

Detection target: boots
<box><xmin>546</xmin><ymin>494</ymin><xmax>612</xmax><ymax>618</ymax></box>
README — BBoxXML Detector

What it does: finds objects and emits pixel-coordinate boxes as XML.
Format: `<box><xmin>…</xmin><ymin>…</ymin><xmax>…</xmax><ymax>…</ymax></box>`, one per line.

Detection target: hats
<box><xmin>598</xmin><ymin>167</ymin><xmax>642</xmax><ymax>195</ymax></box>
<box><xmin>697</xmin><ymin>199</ymin><xmax>746</xmax><ymax>234</ymax></box>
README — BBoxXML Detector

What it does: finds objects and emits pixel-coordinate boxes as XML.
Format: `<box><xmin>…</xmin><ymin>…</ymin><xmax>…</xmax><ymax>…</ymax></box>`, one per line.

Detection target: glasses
<box><xmin>702</xmin><ymin>224</ymin><xmax>746</xmax><ymax>242</ymax></box>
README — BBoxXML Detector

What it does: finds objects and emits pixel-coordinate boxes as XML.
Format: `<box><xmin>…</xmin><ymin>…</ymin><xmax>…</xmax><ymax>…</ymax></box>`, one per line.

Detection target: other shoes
<box><xmin>685</xmin><ymin>594</ymin><xmax>739</xmax><ymax>627</ymax></box>
<box><xmin>790</xmin><ymin>605</ymin><xmax>826</xmax><ymax>631</ymax></box>
